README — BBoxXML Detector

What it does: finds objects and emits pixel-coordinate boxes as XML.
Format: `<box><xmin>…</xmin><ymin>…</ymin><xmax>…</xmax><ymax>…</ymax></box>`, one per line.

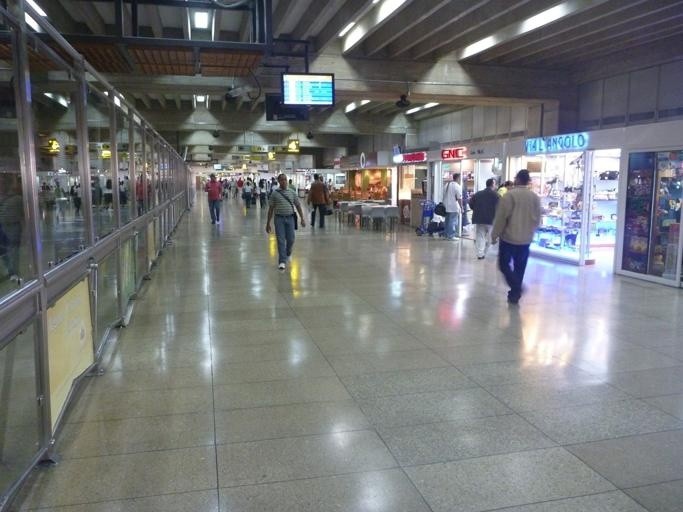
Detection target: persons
<box><xmin>438</xmin><ymin>172</ymin><xmax>465</xmax><ymax>242</ymax></box>
<box><xmin>306</xmin><ymin>173</ymin><xmax>332</xmax><ymax>229</ymax></box>
<box><xmin>326</xmin><ymin>179</ymin><xmax>333</xmax><ymax>193</ymax></box>
<box><xmin>496</xmin><ymin>180</ymin><xmax>514</xmax><ymax>198</ymax></box>
<box><xmin>467</xmin><ymin>178</ymin><xmax>501</xmax><ymax>259</ymax></box>
<box><xmin>264</xmin><ymin>174</ymin><xmax>306</xmax><ymax>269</ymax></box>
<box><xmin>201</xmin><ymin>173</ymin><xmax>297</xmax><ymax>225</ymax></box>
<box><xmin>0</xmin><ymin>174</ymin><xmax>25</xmax><ymax>284</ymax></box>
<box><xmin>489</xmin><ymin>168</ymin><xmax>541</xmax><ymax>305</ymax></box>
<box><xmin>37</xmin><ymin>172</ymin><xmax>153</xmax><ymax>223</ymax></box>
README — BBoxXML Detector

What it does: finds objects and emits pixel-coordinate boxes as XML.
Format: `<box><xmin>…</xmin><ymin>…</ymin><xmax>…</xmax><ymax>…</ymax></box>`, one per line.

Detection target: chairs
<box><xmin>333</xmin><ymin>200</ymin><xmax>400</xmax><ymax>234</ymax></box>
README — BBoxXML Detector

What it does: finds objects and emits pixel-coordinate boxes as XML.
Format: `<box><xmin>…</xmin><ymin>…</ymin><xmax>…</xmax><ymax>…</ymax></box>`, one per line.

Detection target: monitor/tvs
<box><xmin>280</xmin><ymin>72</ymin><xmax>336</xmax><ymax>107</ymax></box>
<box><xmin>266</xmin><ymin>94</ymin><xmax>311</xmax><ymax>123</ymax></box>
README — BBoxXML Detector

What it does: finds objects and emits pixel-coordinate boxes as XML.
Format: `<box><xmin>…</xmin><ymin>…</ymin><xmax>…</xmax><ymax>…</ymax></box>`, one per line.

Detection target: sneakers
<box><xmin>277</xmin><ymin>255</ymin><xmax>294</xmax><ymax>269</ymax></box>
<box><xmin>310</xmin><ymin>222</ymin><xmax>325</xmax><ymax>228</ymax></box>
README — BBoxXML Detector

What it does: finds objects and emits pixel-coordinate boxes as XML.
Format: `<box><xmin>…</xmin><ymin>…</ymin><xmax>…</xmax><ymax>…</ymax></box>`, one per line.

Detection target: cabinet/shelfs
<box><xmin>513</xmin><ymin>156</ymin><xmax>621</xmax><ymax>249</ymax></box>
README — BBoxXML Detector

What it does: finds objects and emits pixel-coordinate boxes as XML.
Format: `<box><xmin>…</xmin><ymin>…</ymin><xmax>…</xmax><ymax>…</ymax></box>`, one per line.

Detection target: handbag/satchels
<box><xmin>292</xmin><ymin>210</ymin><xmax>299</xmax><ymax>231</ymax></box>
<box><xmin>506</xmin><ymin>280</ymin><xmax>525</xmax><ymax>304</ymax></box>
<box><xmin>325</xmin><ymin>208</ymin><xmax>333</xmax><ymax>216</ymax></box>
<box><xmin>434</xmin><ymin>201</ymin><xmax>446</xmax><ymax>217</ymax></box>
